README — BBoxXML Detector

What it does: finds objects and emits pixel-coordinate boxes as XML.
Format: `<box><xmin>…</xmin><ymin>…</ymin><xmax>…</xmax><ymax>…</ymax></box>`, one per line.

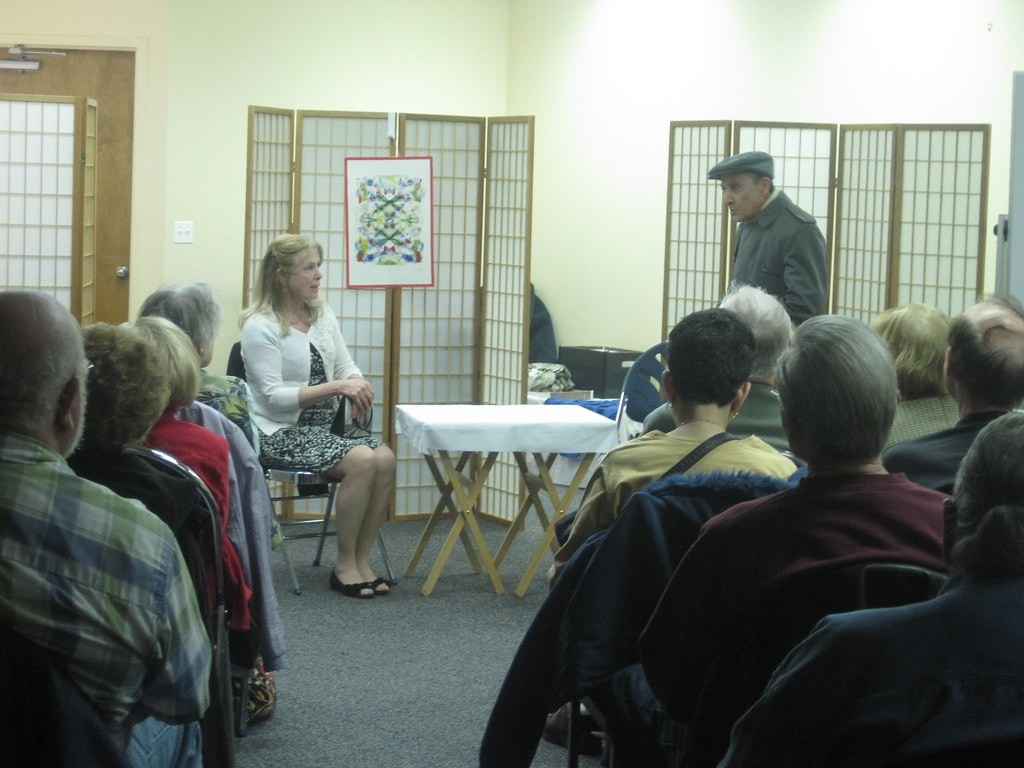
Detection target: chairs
<box><xmin>673</xmin><ymin>564</ymin><xmax>950</xmax><ymax>768</ymax></box>
<box><xmin>227</xmin><ymin>341</ymin><xmax>397</xmax><ymax>595</ymax></box>
<box><xmin>615</xmin><ymin>341</ymin><xmax>668</xmax><ymax>442</ymax></box>
<box><xmin>567</xmin><ymin>474</ymin><xmax>798</xmax><ymax>768</ymax></box>
<box><xmin>143</xmin><ymin>444</ymin><xmax>238</xmax><ymax>768</ymax></box>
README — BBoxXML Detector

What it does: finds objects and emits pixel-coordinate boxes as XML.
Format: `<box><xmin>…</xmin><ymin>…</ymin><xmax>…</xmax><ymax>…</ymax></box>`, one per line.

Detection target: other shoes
<box><xmin>328</xmin><ymin>566</ymin><xmax>375</xmax><ymax>598</ymax></box>
<box><xmin>373</xmin><ymin>576</ymin><xmax>392</xmax><ymax>596</ymax></box>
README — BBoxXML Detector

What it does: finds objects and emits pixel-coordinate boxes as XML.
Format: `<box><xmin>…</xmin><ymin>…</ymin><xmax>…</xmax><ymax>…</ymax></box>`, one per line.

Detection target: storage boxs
<box><xmin>562</xmin><ymin>344</ymin><xmax>642</xmax><ymax>398</ymax></box>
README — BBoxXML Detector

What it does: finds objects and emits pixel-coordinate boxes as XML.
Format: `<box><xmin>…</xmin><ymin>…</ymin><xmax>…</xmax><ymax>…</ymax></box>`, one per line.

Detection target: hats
<box><xmin>707</xmin><ymin>151</ymin><xmax>774</xmax><ymax>181</ymax></box>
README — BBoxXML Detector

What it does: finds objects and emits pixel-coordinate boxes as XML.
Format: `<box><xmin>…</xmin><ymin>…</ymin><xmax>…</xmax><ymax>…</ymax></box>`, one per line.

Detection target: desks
<box><xmin>393</xmin><ymin>404</ymin><xmax>622</xmax><ymax>596</ymax></box>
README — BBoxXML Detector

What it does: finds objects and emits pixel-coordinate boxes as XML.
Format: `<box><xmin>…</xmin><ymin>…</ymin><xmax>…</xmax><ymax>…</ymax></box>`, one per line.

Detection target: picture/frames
<box><xmin>344</xmin><ymin>156</ymin><xmax>435</xmax><ymax>289</ymax></box>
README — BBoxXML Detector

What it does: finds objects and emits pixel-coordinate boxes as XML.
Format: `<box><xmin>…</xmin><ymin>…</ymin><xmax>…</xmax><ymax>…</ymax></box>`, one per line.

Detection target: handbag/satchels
<box><xmin>232</xmin><ymin>664</ymin><xmax>277</xmax><ymax>726</ymax></box>
<box><xmin>329</xmin><ymin>397</ymin><xmax>373</xmax><ymax>438</ymax></box>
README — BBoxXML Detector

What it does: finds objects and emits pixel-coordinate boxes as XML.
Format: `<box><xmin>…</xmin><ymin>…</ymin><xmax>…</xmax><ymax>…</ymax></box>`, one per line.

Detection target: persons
<box><xmin>638</xmin><ymin>313</ymin><xmax>953</xmax><ymax>726</ymax></box>
<box><xmin>67</xmin><ymin>323</ymin><xmax>174</xmax><ymax>447</ymax></box>
<box><xmin>710</xmin><ymin>150</ymin><xmax>828</xmax><ymax>330</ymax></box>
<box><xmin>529</xmin><ymin>281</ymin><xmax>560</xmax><ymax>364</ymax></box>
<box><xmin>235</xmin><ymin>233</ymin><xmax>396</xmax><ymax>599</ymax></box>
<box><xmin>871</xmin><ymin>306</ymin><xmax>960</xmax><ymax>466</ymax></box>
<box><xmin>138</xmin><ymin>279</ymin><xmax>288</xmax><ymax>736</ymax></box>
<box><xmin>634</xmin><ymin>283</ymin><xmax>796</xmax><ymax>457</ymax></box>
<box><xmin>881</xmin><ymin>294</ymin><xmax>1023</xmax><ymax>495</ymax></box>
<box><xmin>717</xmin><ymin>410</ymin><xmax>1024</xmax><ymax>768</ymax></box>
<box><xmin>538</xmin><ymin>308</ymin><xmax>798</xmax><ymax>756</ymax></box>
<box><xmin>1</xmin><ymin>294</ymin><xmax>216</xmax><ymax>768</ymax></box>
<box><xmin>126</xmin><ymin>317</ymin><xmax>255</xmax><ymax>632</ymax></box>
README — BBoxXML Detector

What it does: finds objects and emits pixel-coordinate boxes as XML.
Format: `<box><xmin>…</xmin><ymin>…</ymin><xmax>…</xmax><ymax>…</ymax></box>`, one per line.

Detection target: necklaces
<box><xmin>675</xmin><ymin>418</ymin><xmax>728</xmax><ymax>431</ymax></box>
